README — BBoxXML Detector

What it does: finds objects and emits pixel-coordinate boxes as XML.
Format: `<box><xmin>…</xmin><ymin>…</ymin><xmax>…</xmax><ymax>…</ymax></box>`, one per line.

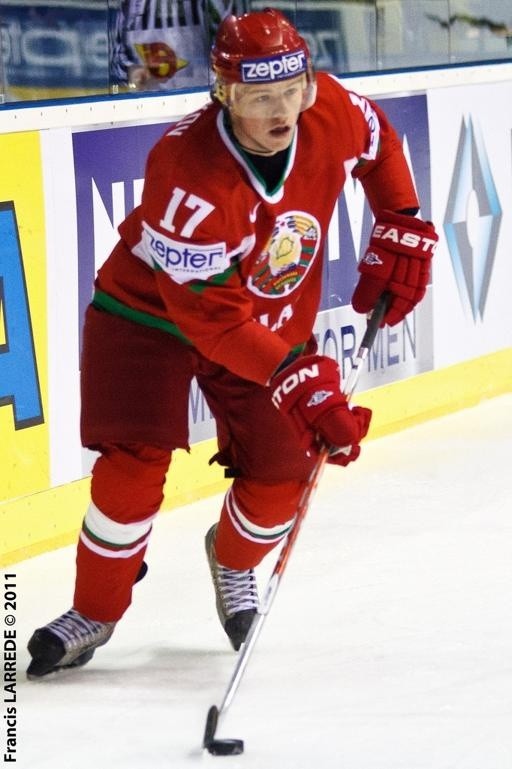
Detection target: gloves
<box><xmin>351</xmin><ymin>209</ymin><xmax>439</xmax><ymax>329</ymax></box>
<box><xmin>268</xmin><ymin>354</ymin><xmax>372</xmax><ymax>466</ymax></box>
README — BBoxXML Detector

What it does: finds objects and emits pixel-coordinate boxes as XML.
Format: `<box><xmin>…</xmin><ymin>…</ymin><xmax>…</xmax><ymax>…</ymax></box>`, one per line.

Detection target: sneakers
<box><xmin>28</xmin><ymin>605</ymin><xmax>117</xmax><ymax>668</ymax></box>
<box><xmin>205</xmin><ymin>522</ymin><xmax>261</xmax><ymax>652</ymax></box>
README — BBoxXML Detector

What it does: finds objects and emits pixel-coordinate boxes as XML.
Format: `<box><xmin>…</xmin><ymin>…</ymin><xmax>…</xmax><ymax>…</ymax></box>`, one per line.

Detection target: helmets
<box><xmin>210</xmin><ymin>6</ymin><xmax>318</xmax><ymax>119</ymax></box>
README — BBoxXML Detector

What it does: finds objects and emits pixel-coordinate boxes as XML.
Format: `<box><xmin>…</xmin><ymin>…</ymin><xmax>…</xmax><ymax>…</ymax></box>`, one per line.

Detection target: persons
<box><xmin>28</xmin><ymin>10</ymin><xmax>440</xmax><ymax>678</ymax></box>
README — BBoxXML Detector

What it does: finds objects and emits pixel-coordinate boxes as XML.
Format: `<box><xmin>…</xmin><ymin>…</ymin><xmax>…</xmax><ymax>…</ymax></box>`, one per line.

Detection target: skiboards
<box><xmin>204</xmin><ymin>303</ymin><xmax>389</xmax><ymax>745</ymax></box>
